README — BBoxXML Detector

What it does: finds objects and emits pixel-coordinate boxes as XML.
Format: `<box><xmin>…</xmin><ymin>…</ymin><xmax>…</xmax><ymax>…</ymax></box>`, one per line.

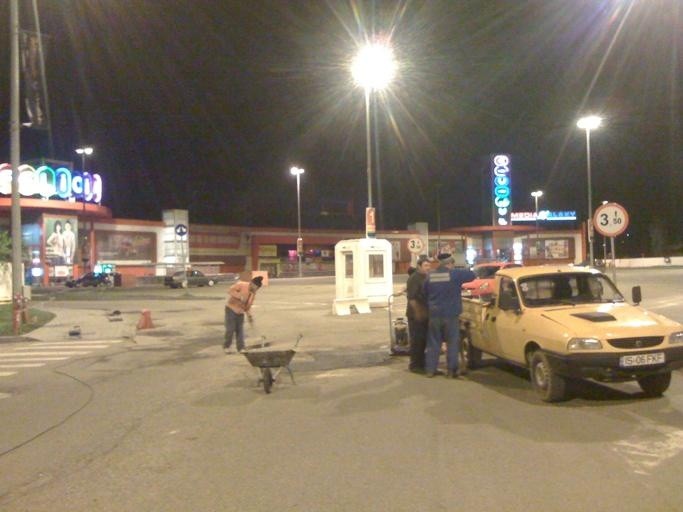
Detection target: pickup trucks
<box><xmin>448</xmin><ymin>266</ymin><xmax>683</xmax><ymax>405</ymax></box>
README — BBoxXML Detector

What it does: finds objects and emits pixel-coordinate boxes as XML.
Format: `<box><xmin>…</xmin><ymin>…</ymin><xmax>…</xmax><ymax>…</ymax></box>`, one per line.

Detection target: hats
<box><xmin>252</xmin><ymin>277</ymin><xmax>263</xmax><ymax>286</ymax></box>
<box><xmin>438</xmin><ymin>254</ymin><xmax>451</xmax><ymax>258</ymax></box>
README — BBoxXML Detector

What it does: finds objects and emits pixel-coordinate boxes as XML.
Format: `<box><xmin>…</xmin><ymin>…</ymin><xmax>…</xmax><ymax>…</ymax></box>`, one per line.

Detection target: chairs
<box><xmin>551</xmin><ymin>279</ymin><xmax>572</xmax><ymax>299</ymax></box>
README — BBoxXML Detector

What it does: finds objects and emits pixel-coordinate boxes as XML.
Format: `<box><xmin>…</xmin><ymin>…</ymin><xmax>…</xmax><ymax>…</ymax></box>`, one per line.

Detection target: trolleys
<box><xmin>386</xmin><ymin>292</ymin><xmax>409</xmax><ymax>353</ymax></box>
<box><xmin>241</xmin><ymin>332</ymin><xmax>305</xmax><ymax>393</ymax></box>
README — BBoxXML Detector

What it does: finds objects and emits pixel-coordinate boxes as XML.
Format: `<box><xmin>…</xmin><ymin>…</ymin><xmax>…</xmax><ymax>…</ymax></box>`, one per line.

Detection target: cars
<box><xmin>165</xmin><ymin>269</ymin><xmax>218</xmax><ymax>289</ymax></box>
<box><xmin>461</xmin><ymin>260</ymin><xmax>524</xmax><ymax>302</ymax></box>
<box><xmin>66</xmin><ymin>272</ymin><xmax>122</xmax><ymax>288</ymax></box>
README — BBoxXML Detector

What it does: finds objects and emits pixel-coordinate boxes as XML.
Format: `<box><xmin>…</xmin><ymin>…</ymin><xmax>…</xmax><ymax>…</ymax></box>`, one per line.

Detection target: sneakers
<box><xmin>411</xmin><ymin>366</ymin><xmax>457</xmax><ymax>378</ymax></box>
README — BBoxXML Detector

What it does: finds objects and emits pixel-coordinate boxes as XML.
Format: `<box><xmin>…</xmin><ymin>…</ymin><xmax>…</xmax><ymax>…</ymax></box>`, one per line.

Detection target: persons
<box><xmin>62</xmin><ymin>221</ymin><xmax>76</xmax><ymax>260</ymax></box>
<box><xmin>223</xmin><ymin>276</ymin><xmax>265</xmax><ymax>355</ymax></box>
<box><xmin>408</xmin><ymin>257</ymin><xmax>432</xmax><ymax>375</ymax></box>
<box><xmin>47</xmin><ymin>221</ymin><xmax>63</xmax><ymax>255</ymax></box>
<box><xmin>422</xmin><ymin>252</ymin><xmax>478</xmax><ymax>379</ymax></box>
<box><xmin>395</xmin><ymin>266</ymin><xmax>415</xmax><ymax>297</ymax></box>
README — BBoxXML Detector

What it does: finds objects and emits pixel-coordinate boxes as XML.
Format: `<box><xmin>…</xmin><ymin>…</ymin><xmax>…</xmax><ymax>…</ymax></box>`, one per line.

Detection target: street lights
<box><xmin>433</xmin><ymin>181</ymin><xmax>444</xmax><ymax>256</ymax></box>
<box><xmin>75</xmin><ymin>146</ymin><xmax>93</xmax><ymax>276</ymax></box>
<box><xmin>577</xmin><ymin>113</ymin><xmax>602</xmax><ymax>269</ymax></box>
<box><xmin>288</xmin><ymin>166</ymin><xmax>305</xmax><ymax>279</ymax></box>
<box><xmin>530</xmin><ymin>191</ymin><xmax>544</xmax><ymax>264</ymax></box>
<box><xmin>350</xmin><ymin>38</ymin><xmax>399</xmax><ymax>240</ymax></box>
